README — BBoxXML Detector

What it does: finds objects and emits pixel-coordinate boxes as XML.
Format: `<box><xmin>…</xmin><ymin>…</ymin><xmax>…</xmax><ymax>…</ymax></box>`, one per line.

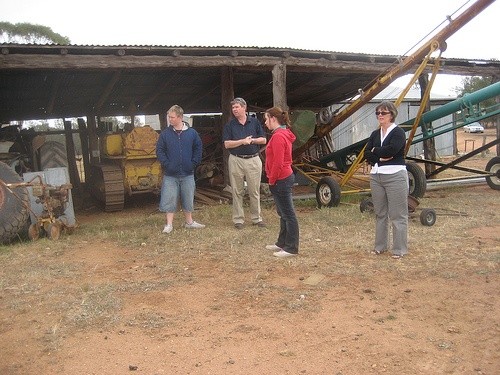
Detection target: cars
<box><xmin>463</xmin><ymin>122</ymin><xmax>485</xmax><ymax>135</ymax></box>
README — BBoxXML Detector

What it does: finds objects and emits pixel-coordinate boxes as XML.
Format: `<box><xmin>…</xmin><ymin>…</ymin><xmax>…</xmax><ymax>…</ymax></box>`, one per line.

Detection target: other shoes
<box><xmin>252</xmin><ymin>221</ymin><xmax>265</xmax><ymax>227</ymax></box>
<box><xmin>235</xmin><ymin>223</ymin><xmax>243</xmax><ymax>229</ymax></box>
<box><xmin>266</xmin><ymin>244</ymin><xmax>282</xmax><ymax>249</ymax></box>
<box><xmin>273</xmin><ymin>249</ymin><xmax>298</xmax><ymax>257</ymax></box>
<box><xmin>392</xmin><ymin>254</ymin><xmax>403</xmax><ymax>259</ymax></box>
<box><xmin>372</xmin><ymin>249</ymin><xmax>387</xmax><ymax>254</ymax></box>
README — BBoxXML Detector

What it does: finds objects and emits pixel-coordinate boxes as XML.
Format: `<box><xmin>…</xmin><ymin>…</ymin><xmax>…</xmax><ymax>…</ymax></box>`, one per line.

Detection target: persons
<box><xmin>265</xmin><ymin>107</ymin><xmax>299</xmax><ymax>257</ymax></box>
<box><xmin>157</xmin><ymin>105</ymin><xmax>205</xmax><ymax>233</ymax></box>
<box><xmin>364</xmin><ymin>101</ymin><xmax>407</xmax><ymax>259</ymax></box>
<box><xmin>224</xmin><ymin>97</ymin><xmax>267</xmax><ymax>230</ymax></box>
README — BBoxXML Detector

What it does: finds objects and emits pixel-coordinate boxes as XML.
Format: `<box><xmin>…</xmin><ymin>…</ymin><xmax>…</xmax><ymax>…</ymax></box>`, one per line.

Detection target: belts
<box><xmin>231</xmin><ymin>153</ymin><xmax>258</xmax><ymax>160</ymax></box>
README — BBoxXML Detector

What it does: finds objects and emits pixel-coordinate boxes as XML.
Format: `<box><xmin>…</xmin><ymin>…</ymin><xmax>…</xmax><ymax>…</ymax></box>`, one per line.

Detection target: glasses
<box><xmin>375</xmin><ymin>111</ymin><xmax>391</xmax><ymax>115</ymax></box>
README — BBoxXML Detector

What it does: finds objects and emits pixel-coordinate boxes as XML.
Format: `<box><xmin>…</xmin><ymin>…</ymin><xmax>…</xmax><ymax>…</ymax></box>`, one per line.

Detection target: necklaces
<box><xmin>172</xmin><ymin>122</ymin><xmax>185</xmax><ymax>135</ymax></box>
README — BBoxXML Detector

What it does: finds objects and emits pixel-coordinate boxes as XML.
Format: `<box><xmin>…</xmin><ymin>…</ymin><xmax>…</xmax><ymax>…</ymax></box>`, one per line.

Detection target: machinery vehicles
<box><xmin>84</xmin><ymin>116</ymin><xmax>167</xmax><ymax>212</ymax></box>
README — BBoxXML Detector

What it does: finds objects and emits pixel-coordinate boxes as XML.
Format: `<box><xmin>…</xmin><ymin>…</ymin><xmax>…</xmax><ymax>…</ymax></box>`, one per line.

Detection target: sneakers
<box><xmin>162</xmin><ymin>223</ymin><xmax>173</xmax><ymax>234</ymax></box>
<box><xmin>185</xmin><ymin>220</ymin><xmax>206</xmax><ymax>228</ymax></box>
